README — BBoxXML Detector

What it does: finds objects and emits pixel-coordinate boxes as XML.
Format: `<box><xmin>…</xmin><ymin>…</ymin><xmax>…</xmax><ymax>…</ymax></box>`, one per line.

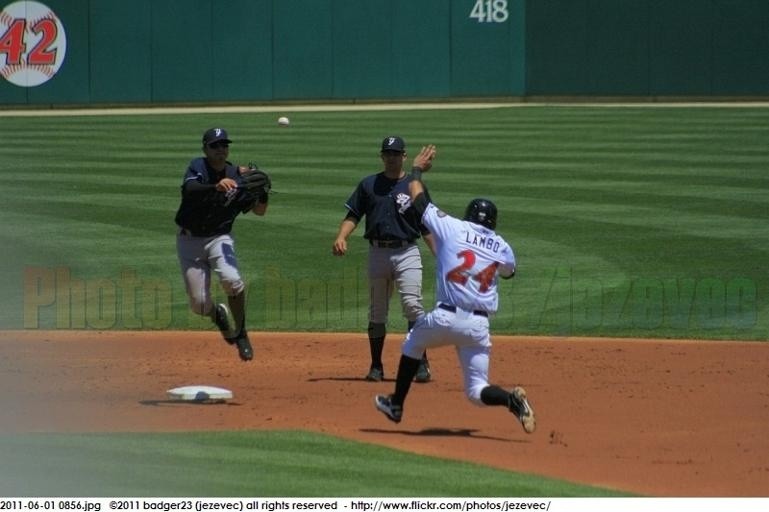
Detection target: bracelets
<box><xmin>410</xmin><ymin>164</ymin><xmax>422</xmax><ymax>184</ymax></box>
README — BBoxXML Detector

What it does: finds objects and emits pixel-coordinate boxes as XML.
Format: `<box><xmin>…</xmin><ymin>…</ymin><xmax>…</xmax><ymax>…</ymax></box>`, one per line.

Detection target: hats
<box><xmin>380</xmin><ymin>136</ymin><xmax>405</xmax><ymax>153</ymax></box>
<box><xmin>202</xmin><ymin>128</ymin><xmax>232</xmax><ymax>147</ymax></box>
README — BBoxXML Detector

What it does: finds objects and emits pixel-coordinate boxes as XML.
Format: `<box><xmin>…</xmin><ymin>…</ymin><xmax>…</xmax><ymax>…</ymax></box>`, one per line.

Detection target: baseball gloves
<box><xmin>225</xmin><ymin>171</ymin><xmax>271</xmax><ymax>213</ymax></box>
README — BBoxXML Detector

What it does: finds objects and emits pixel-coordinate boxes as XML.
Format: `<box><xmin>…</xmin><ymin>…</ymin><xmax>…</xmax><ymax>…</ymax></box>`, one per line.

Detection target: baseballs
<box><xmin>278</xmin><ymin>117</ymin><xmax>289</xmax><ymax>126</ymax></box>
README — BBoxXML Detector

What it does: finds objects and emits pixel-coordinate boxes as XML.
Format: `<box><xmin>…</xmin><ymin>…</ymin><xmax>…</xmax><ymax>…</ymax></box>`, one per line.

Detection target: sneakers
<box><xmin>237</xmin><ymin>336</ymin><xmax>253</xmax><ymax>361</ymax></box>
<box><xmin>508</xmin><ymin>386</ymin><xmax>537</xmax><ymax>434</ymax></box>
<box><xmin>215</xmin><ymin>303</ymin><xmax>235</xmax><ymax>346</ymax></box>
<box><xmin>374</xmin><ymin>394</ymin><xmax>402</xmax><ymax>424</ymax></box>
<box><xmin>366</xmin><ymin>364</ymin><xmax>384</xmax><ymax>382</ymax></box>
<box><xmin>415</xmin><ymin>360</ymin><xmax>431</xmax><ymax>382</ymax></box>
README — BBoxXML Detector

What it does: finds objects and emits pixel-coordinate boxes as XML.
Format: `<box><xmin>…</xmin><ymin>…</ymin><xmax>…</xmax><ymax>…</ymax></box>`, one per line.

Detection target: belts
<box><xmin>371</xmin><ymin>240</ymin><xmax>410</xmax><ymax>248</ymax></box>
<box><xmin>439</xmin><ymin>303</ymin><xmax>488</xmax><ymax>318</ymax></box>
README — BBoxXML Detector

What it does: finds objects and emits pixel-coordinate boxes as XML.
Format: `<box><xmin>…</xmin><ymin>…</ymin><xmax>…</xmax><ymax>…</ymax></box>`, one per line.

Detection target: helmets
<box><xmin>465</xmin><ymin>198</ymin><xmax>497</xmax><ymax>227</ymax></box>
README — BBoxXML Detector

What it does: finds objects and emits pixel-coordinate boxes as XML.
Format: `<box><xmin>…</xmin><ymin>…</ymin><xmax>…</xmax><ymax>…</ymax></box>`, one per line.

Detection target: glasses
<box><xmin>385</xmin><ymin>151</ymin><xmax>400</xmax><ymax>157</ymax></box>
<box><xmin>210</xmin><ymin>144</ymin><xmax>229</xmax><ymax>149</ymax></box>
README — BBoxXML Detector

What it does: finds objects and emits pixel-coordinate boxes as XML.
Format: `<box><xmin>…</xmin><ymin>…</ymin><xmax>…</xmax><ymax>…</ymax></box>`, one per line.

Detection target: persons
<box><xmin>372</xmin><ymin>143</ymin><xmax>538</xmax><ymax>433</ymax></box>
<box><xmin>172</xmin><ymin>127</ymin><xmax>272</xmax><ymax>363</ymax></box>
<box><xmin>332</xmin><ymin>134</ymin><xmax>438</xmax><ymax>388</ymax></box>
